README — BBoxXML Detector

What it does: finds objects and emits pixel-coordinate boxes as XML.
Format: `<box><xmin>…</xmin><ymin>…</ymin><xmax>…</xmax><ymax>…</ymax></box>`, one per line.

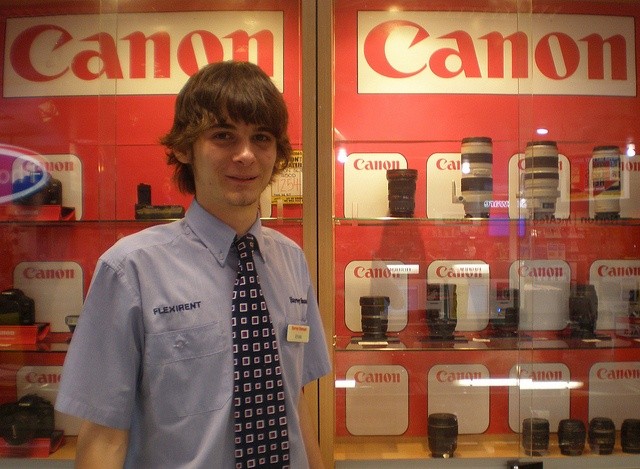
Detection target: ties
<box><xmin>230</xmin><ymin>234</ymin><xmax>291</xmax><ymax>469</ymax></box>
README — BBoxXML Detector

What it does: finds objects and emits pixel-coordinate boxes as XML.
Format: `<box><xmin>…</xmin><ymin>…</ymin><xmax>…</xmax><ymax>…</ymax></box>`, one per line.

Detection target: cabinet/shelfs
<box><xmin>0</xmin><ymin>210</ymin><xmax>318</xmax><ymax>469</ymax></box>
<box><xmin>317</xmin><ymin>213</ymin><xmax>639</xmax><ymax>468</ymax></box>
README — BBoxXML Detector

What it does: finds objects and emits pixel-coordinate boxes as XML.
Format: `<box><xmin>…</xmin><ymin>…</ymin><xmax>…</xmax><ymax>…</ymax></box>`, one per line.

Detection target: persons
<box><xmin>54</xmin><ymin>59</ymin><xmax>326</xmax><ymax>469</ymax></box>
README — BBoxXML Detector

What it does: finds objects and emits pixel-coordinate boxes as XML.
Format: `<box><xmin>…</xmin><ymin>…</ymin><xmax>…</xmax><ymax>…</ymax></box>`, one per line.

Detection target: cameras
<box><xmin>480</xmin><ymin>288</ymin><xmax>533</xmax><ymax>342</ymax></box>
<box><xmin>1</xmin><ymin>394</ymin><xmax>54</xmax><ymax>445</ymax></box>
<box><xmin>12</xmin><ymin>170</ymin><xmax>62</xmax><ymax>206</ymax></box>
<box><xmin>452</xmin><ymin>136</ymin><xmax>494</xmax><ymax>218</ymax></box>
<box><xmin>621</xmin><ymin>419</ymin><xmax>640</xmax><ymax>453</ymax></box>
<box><xmin>135</xmin><ymin>182</ymin><xmax>184</xmax><ymax>219</ymax></box>
<box><xmin>386</xmin><ymin>168</ymin><xmax>419</xmax><ymax>218</ymax></box>
<box><xmin>416</xmin><ymin>284</ymin><xmax>470</xmax><ymax>342</ymax></box>
<box><xmin>558</xmin><ymin>420</ymin><xmax>586</xmax><ymax>455</ymax></box>
<box><xmin>588</xmin><ymin>417</ymin><xmax>616</xmax><ymax>454</ymax></box>
<box><xmin>426</xmin><ymin>412</ymin><xmax>458</xmax><ymax>458</ymax></box>
<box><xmin>522</xmin><ymin>418</ymin><xmax>550</xmax><ymax>457</ymax></box>
<box><xmin>625</xmin><ymin>289</ymin><xmax>640</xmax><ymax>338</ymax></box>
<box><xmin>517</xmin><ymin>140</ymin><xmax>561</xmax><ymax>220</ymax></box>
<box><xmin>561</xmin><ymin>284</ymin><xmax>610</xmax><ymax>341</ymax></box>
<box><xmin>591</xmin><ymin>145</ymin><xmax>623</xmax><ymax>220</ymax></box>
<box><xmin>1</xmin><ymin>288</ymin><xmax>36</xmax><ymax>325</ymax></box>
<box><xmin>352</xmin><ymin>295</ymin><xmax>400</xmax><ymax>344</ymax></box>
